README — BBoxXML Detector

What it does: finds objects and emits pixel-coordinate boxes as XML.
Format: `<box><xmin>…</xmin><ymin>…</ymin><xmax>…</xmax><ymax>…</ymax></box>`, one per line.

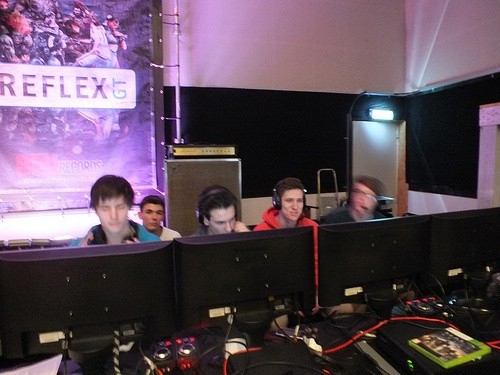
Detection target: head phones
<box><xmin>94</xmin><ymin>220</ymin><xmax>139</xmax><ymax>245</ymax></box>
<box><xmin>195</xmin><ymin>189</ymin><xmax>230</xmax><ymax>223</ymax></box>
<box><xmin>272</xmin><ymin>183</ymin><xmax>306</xmax><ymax>210</ymax></box>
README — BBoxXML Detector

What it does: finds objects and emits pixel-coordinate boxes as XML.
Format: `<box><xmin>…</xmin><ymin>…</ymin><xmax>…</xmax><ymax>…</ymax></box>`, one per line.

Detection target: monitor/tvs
<box><xmin>317</xmin><ymin>215</ymin><xmax>432</xmax><ymax>307</ymax></box>
<box><xmin>174</xmin><ymin>226</ymin><xmax>316</xmax><ymax>329</ymax></box>
<box><xmin>432</xmin><ymin>207</ymin><xmax>500</xmax><ymax>282</ymax></box>
<box><xmin>0</xmin><ymin>241</ymin><xmax>181</xmax><ymax>360</ymax></box>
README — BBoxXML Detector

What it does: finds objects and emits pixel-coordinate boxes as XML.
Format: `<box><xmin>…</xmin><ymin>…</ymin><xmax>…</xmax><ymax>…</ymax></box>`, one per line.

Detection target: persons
<box><xmin>137</xmin><ymin>195</ymin><xmax>182</xmax><ymax>242</ymax></box>
<box><xmin>253</xmin><ymin>178</ymin><xmax>320</xmax><ymax>286</ymax></box>
<box><xmin>323</xmin><ymin>176</ymin><xmax>386</xmax><ymax>223</ymax></box>
<box><xmin>69</xmin><ymin>175</ymin><xmax>161</xmax><ymax>247</ymax></box>
<box><xmin>190</xmin><ymin>185</ymin><xmax>249</xmax><ymax>236</ymax></box>
